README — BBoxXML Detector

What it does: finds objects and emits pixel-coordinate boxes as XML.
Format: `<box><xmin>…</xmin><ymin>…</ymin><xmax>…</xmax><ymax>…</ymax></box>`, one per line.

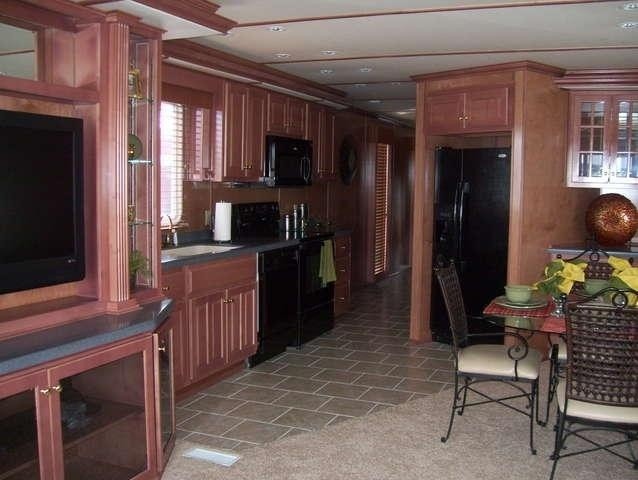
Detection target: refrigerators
<box><xmin>430</xmin><ymin>146</ymin><xmax>512</xmax><ymax>347</ymax></box>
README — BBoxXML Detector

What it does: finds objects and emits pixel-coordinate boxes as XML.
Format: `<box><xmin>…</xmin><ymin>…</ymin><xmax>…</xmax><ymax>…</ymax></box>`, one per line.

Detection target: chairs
<box><xmin>548</xmin><ymin>287</ymin><xmax>637</xmax><ymax>480</ymax></box>
<box><xmin>434</xmin><ymin>256</ymin><xmax>543</xmax><ymax>456</ymax></box>
<box><xmin>543</xmin><ymin>244</ymin><xmax>633</xmax><ymax>402</ymax></box>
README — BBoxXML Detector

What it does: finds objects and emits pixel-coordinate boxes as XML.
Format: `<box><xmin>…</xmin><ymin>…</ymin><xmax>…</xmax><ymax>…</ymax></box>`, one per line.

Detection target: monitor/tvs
<box><xmin>0</xmin><ymin>108</ymin><xmax>86</xmax><ymax>297</ymax></box>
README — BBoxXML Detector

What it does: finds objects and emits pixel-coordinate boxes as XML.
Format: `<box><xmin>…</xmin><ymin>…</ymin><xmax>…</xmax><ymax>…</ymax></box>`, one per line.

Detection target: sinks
<box><xmin>162</xmin><ymin>243</ymin><xmax>241</xmax><ymax>263</ymax></box>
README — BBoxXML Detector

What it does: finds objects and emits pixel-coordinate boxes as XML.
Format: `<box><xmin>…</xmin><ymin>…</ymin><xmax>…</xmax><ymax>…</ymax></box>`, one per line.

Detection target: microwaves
<box><xmin>213</xmin><ymin>201</ymin><xmax>281</xmax><ymax>245</ymax></box>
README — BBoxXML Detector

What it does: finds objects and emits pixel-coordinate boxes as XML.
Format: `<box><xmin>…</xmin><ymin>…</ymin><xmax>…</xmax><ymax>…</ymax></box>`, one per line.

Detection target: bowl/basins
<box><xmin>504</xmin><ymin>286</ymin><xmax>537</xmax><ymax>303</ymax></box>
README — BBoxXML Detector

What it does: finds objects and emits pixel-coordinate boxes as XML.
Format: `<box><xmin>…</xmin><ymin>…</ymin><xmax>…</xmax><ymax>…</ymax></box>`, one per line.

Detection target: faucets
<box><xmin>161</xmin><ymin>213</ymin><xmax>174</xmax><ymax>235</ymax></box>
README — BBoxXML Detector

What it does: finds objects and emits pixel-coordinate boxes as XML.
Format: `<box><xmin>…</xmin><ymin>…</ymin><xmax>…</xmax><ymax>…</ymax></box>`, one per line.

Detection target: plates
<box><xmin>493</xmin><ymin>296</ymin><xmax>549</xmax><ymax>309</ymax></box>
<box><xmin>502</xmin><ymin>300</ymin><xmax>542</xmax><ymax>306</ymax></box>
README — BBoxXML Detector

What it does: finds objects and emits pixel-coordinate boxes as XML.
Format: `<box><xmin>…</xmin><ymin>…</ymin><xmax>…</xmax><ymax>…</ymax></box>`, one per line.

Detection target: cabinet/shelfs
<box><xmin>189</xmin><ymin>255</ymin><xmax>258</xmax><ymax>397</ymax></box>
<box><xmin>265</xmin><ymin>88</ymin><xmax>307</xmax><ymax>138</ymax></box>
<box><xmin>1</xmin><ymin>334</ymin><xmax>155</xmax><ymax>480</ymax></box>
<box><xmin>424</xmin><ymin>85</ymin><xmax>512</xmax><ymax>134</ymax></box>
<box><xmin>563</xmin><ymin>90</ymin><xmax>638</xmax><ymax>191</ymax></box>
<box><xmin>108</xmin><ymin>10</ymin><xmax>160</xmax><ymax>315</ymax></box>
<box><xmin>314</xmin><ymin>105</ymin><xmax>333</xmax><ymax>176</ymax></box>
<box><xmin>223</xmin><ymin>81</ymin><xmax>264</xmax><ymax>184</ymax></box>
<box><xmin>334</xmin><ymin>234</ymin><xmax>351</xmax><ymax>322</ymax></box>
<box><xmin>154</xmin><ymin>306</ymin><xmax>173</xmax><ymax>480</ymax></box>
<box><xmin>0</xmin><ymin>0</ymin><xmax>107</xmax><ymax>379</ymax></box>
<box><xmin>247</xmin><ymin>236</ymin><xmax>338</xmax><ymax>365</ymax></box>
<box><xmin>163</xmin><ymin>269</ymin><xmax>187</xmax><ymax>403</ymax></box>
